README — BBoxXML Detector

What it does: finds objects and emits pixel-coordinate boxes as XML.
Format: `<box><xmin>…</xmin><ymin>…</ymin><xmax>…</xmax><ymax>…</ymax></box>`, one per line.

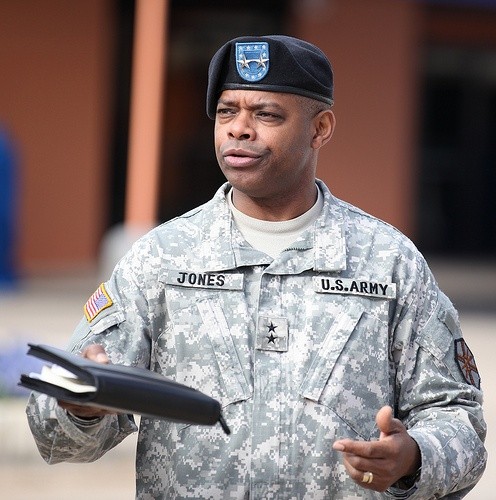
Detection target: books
<box><xmin>18</xmin><ymin>344</ymin><xmax>231</xmax><ymax>435</ymax></box>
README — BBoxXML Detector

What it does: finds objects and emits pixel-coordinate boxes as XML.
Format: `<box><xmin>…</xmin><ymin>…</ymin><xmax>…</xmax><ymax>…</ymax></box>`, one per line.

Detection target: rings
<box><xmin>360</xmin><ymin>472</ymin><xmax>374</xmax><ymax>485</ymax></box>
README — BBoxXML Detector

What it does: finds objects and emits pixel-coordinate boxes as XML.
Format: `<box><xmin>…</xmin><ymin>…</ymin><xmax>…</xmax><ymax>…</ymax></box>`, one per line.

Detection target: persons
<box><xmin>20</xmin><ymin>33</ymin><xmax>487</xmax><ymax>497</ymax></box>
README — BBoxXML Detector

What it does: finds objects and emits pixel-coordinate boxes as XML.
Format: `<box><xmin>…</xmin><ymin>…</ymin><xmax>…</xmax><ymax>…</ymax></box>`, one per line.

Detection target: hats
<box><xmin>205</xmin><ymin>35</ymin><xmax>332</xmax><ymax>120</ymax></box>
